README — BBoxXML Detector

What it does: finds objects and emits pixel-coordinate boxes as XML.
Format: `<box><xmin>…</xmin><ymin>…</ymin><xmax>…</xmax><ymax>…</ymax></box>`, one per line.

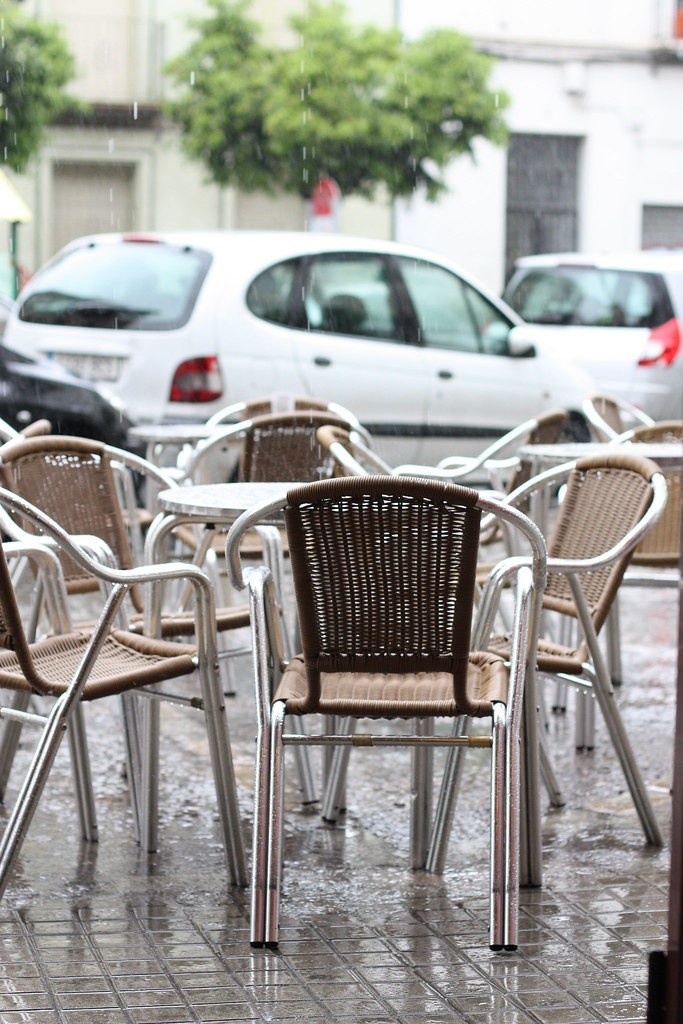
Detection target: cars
<box><xmin>476</xmin><ymin>251</ymin><xmax>683</xmax><ymax>455</ymax></box>
<box><xmin>0</xmin><ymin>227</ymin><xmax>607</xmax><ymax>513</ymax></box>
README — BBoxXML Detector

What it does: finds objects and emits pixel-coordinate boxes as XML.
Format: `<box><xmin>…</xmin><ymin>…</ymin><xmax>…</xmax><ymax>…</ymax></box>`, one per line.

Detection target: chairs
<box><xmin>0</xmin><ymin>393</ymin><xmax>683</xmax><ymax>952</ymax></box>
<box><xmin>321</xmin><ymin>293</ymin><xmax>367</xmax><ymax>331</ymax></box>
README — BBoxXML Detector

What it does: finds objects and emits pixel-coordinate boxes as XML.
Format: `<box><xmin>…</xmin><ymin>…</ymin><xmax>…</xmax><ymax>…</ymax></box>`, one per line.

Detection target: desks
<box><xmin>141</xmin><ymin>481</ymin><xmax>504</xmax><ymax>854</ymax></box>
<box><xmin>523</xmin><ymin>440</ymin><xmax>682</xmax><ymax>542</ymax></box>
